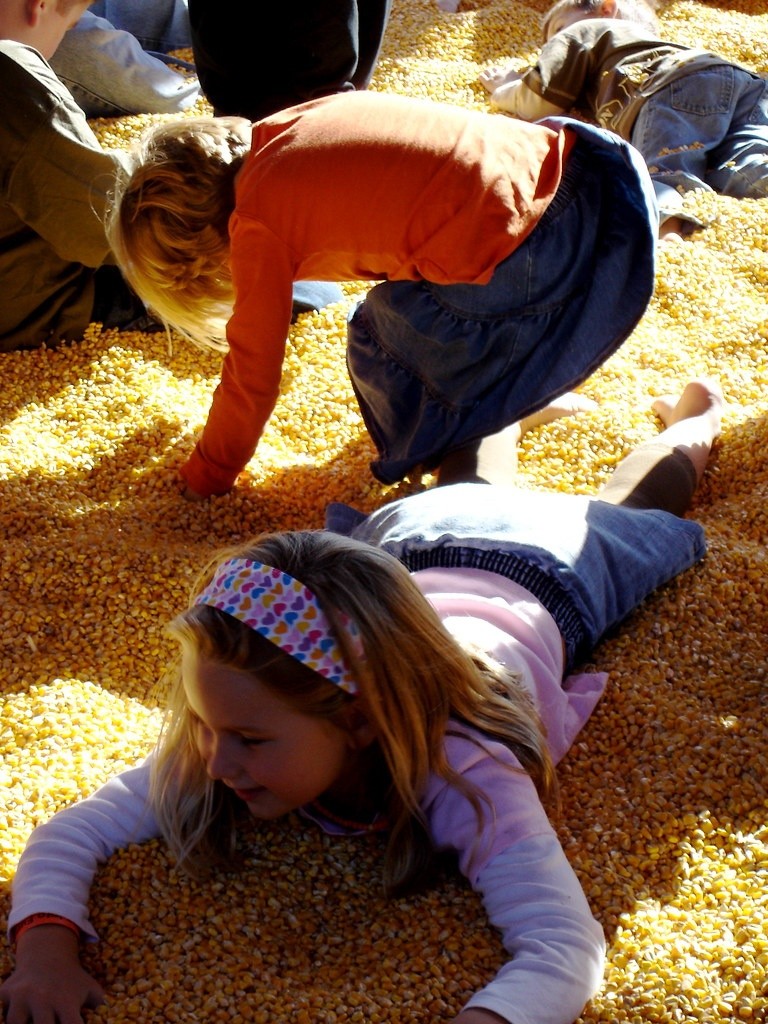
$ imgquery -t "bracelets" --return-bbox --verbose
[16,912,79,940]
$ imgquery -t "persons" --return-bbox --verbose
[478,0,768,244]
[0,380,736,1024]
[0,0,390,350]
[108,91,661,500]
